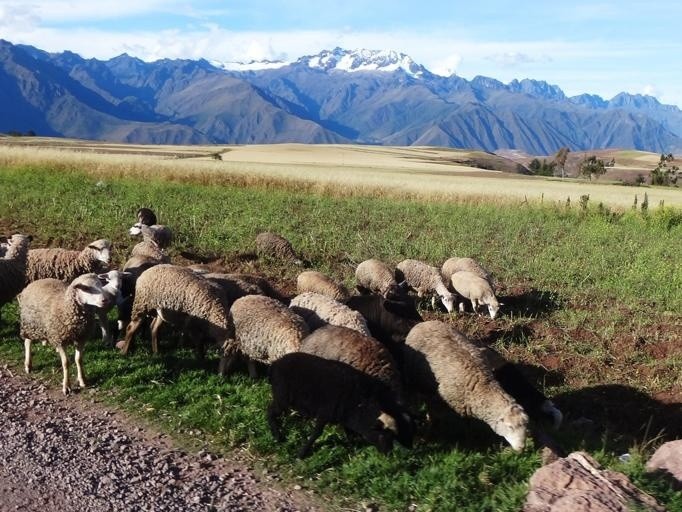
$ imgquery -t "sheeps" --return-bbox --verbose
[1,237,114,288]
[257,234,305,267]
[441,255,490,288]
[298,324,415,447]
[289,291,371,337]
[129,222,175,250]
[229,293,308,379]
[1,233,30,307]
[16,273,107,395]
[137,205,159,227]
[132,241,166,263]
[123,254,161,306]
[297,271,350,304]
[449,269,504,322]
[204,272,274,296]
[401,318,531,458]
[395,255,459,315]
[264,350,400,459]
[119,262,241,378]
[347,294,401,356]
[85,270,123,345]
[353,259,402,300]
